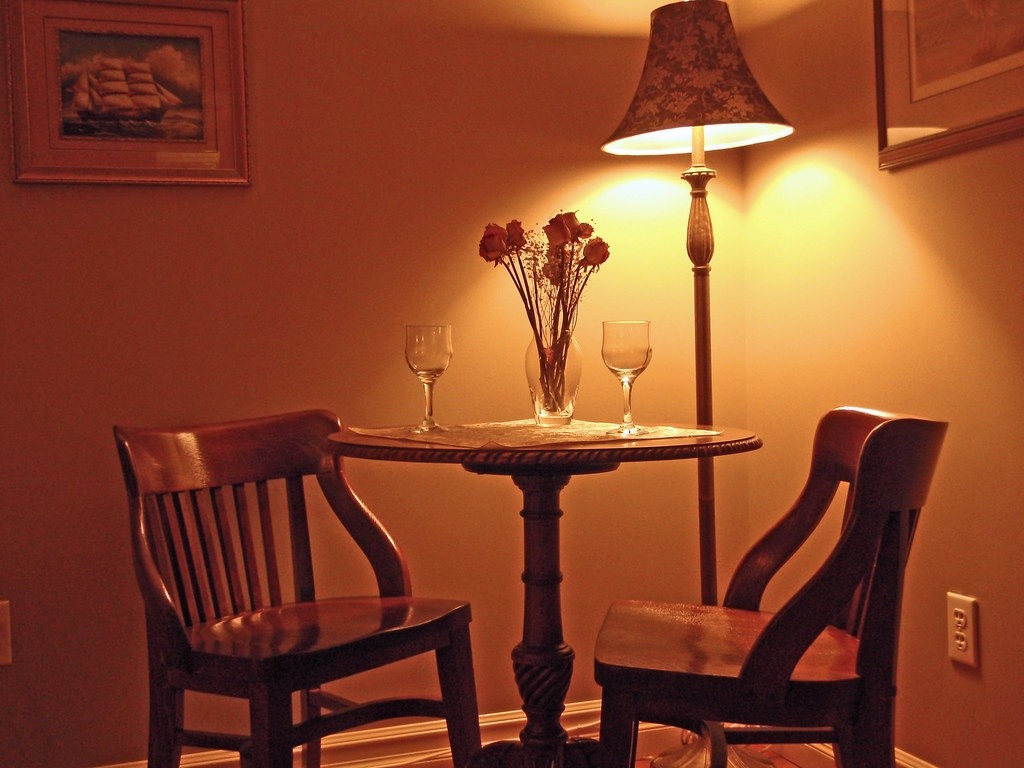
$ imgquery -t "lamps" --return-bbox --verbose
[601,0,795,768]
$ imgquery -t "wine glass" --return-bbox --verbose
[405,324,454,435]
[601,320,653,437]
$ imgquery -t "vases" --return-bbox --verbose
[524,327,585,425]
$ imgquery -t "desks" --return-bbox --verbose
[325,425,764,768]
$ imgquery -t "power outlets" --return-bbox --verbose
[946,590,976,670]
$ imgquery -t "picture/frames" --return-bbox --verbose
[0,0,250,187]
[873,0,1023,169]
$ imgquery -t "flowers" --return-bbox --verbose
[477,209,609,410]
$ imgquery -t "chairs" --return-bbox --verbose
[594,407,950,768]
[114,408,481,768]
